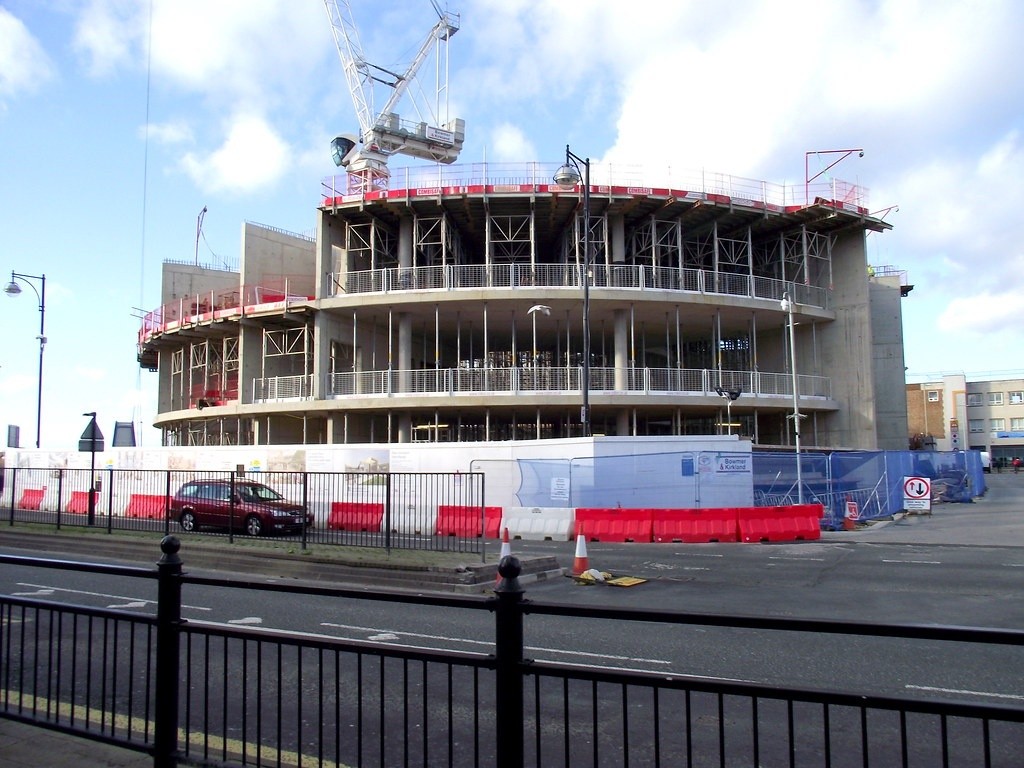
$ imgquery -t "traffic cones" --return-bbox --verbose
[838,495,859,531]
[495,526,511,584]
[565,521,591,577]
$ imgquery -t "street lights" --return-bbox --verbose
[82,411,97,526]
[551,142,590,437]
[923,382,933,436]
[781,290,805,505]
[714,386,742,435]
[3,269,45,448]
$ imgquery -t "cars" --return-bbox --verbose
[170,478,315,536]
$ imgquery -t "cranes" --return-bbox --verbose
[320,0,467,195]
